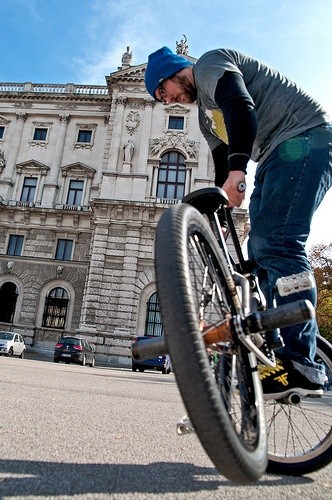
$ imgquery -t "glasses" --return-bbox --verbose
[159,80,170,106]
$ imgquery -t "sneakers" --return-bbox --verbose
[235,358,323,400]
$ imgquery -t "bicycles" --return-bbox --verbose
[135,180,332,483]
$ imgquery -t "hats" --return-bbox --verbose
[144,46,195,102]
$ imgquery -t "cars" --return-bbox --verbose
[131,334,172,375]
[53,336,96,367]
[0,331,26,359]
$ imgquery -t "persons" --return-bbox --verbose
[146,46,332,397]
[123,140,135,162]
[121,33,189,67]
